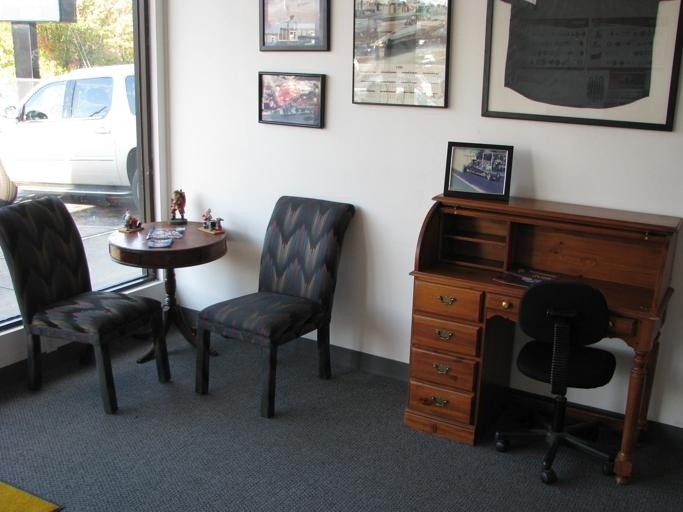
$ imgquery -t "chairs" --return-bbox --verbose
[195,195,354,419]
[0,198,170,414]
[495,281,616,484]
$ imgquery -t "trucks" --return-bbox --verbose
[463,152,505,181]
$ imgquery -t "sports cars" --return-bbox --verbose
[359,14,444,52]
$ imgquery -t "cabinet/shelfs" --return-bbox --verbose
[403,193,682,485]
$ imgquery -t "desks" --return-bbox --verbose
[108,221,228,363]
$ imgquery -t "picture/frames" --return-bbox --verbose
[352,0,451,108]
[258,71,325,128]
[259,0,330,51]
[481,0,683,131]
[443,141,514,201]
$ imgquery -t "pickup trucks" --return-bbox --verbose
[0,64,142,214]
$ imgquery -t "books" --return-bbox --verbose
[491,266,557,290]
[145,225,186,240]
